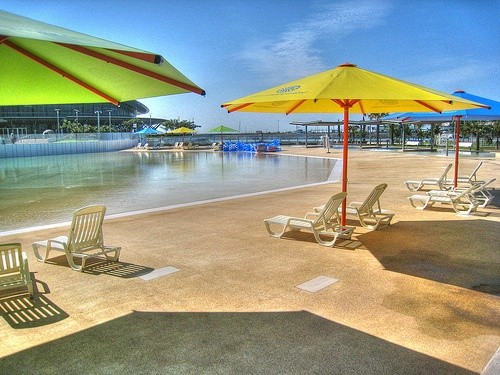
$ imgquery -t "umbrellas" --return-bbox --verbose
[132,127,162,146]
[221,62,490,231]
[379,91,500,189]
[165,127,199,144]
[207,125,240,143]
[0,10,206,108]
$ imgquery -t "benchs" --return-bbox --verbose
[454,142,473,150]
[404,141,419,148]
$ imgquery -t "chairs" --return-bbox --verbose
[404,162,496,215]
[132,142,183,150]
[262,192,355,247]
[32,205,121,272]
[305,183,395,231]
[0,243,34,300]
